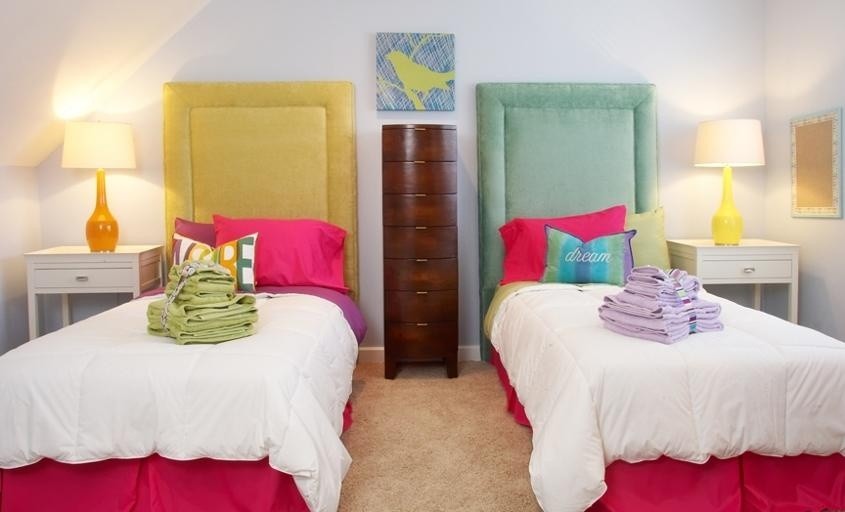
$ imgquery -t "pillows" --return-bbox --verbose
[162,228,263,296]
[209,209,353,296]
[494,198,630,287]
[533,220,640,289]
[170,214,215,246]
[622,203,673,272]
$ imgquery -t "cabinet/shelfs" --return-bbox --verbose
[376,121,464,384]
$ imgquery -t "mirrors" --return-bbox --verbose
[786,104,843,224]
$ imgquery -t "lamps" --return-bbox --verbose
[57,115,145,254]
[691,115,767,246]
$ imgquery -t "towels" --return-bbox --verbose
[138,257,262,349]
[596,260,726,348]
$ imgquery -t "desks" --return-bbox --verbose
[662,233,802,326]
[21,242,162,343]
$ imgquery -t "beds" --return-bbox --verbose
[472,80,845,510]
[0,75,364,512]
